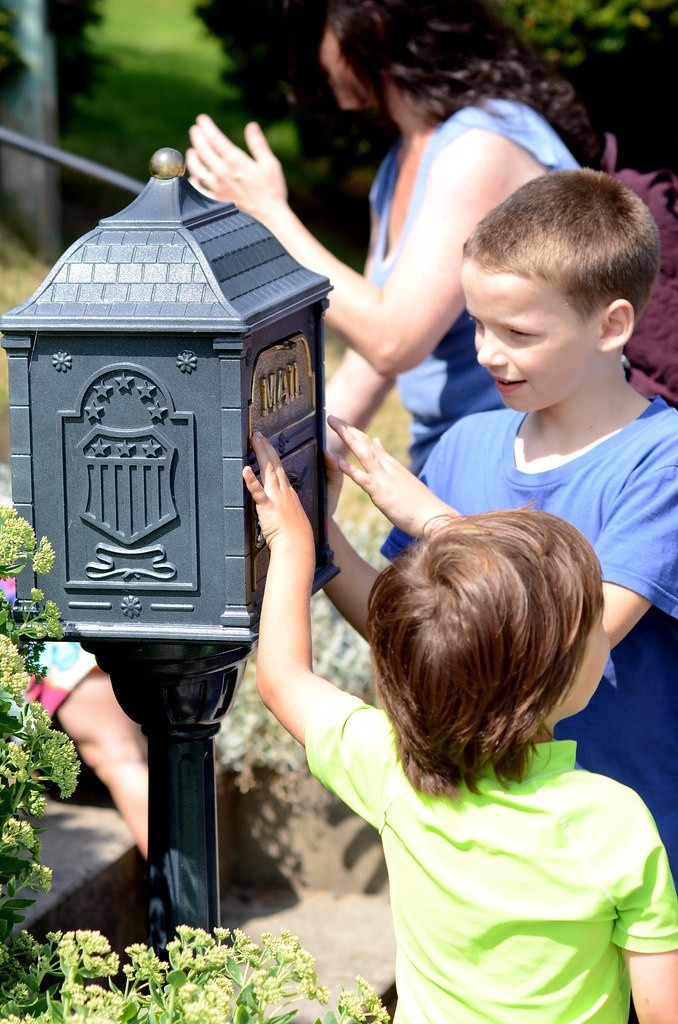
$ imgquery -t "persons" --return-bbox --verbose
[322,169,678,895]
[186,0,602,477]
[240,427,678,1024]
[0,345,149,986]
